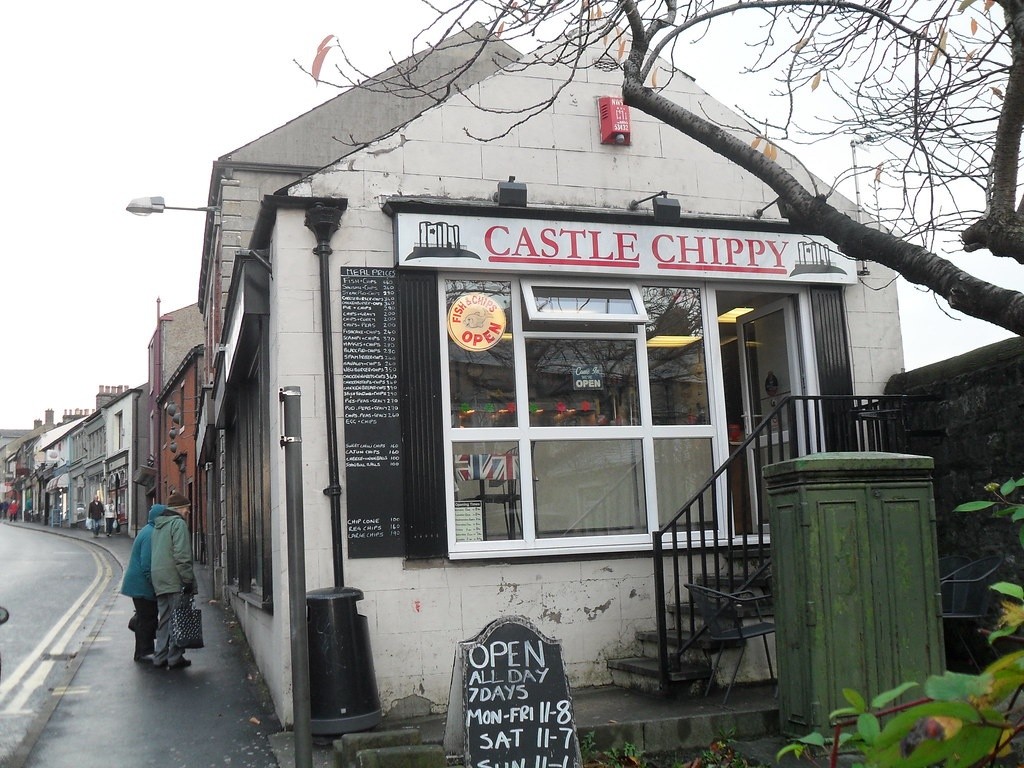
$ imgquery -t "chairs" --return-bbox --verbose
[683,583,776,704]
[938,555,1005,675]
[476,442,535,538]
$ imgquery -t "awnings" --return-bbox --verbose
[46,473,68,494]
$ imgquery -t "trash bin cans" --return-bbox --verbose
[307,585,382,734]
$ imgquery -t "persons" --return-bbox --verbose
[9,499,17,521]
[88,495,105,537]
[151,491,198,668]
[120,504,169,663]
[104,498,117,537]
[2,500,9,519]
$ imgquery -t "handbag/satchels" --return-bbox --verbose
[112,519,117,528]
[171,586,204,650]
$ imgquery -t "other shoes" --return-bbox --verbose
[107,532,111,537]
[168,658,191,671]
[128,622,137,632]
[134,652,153,664]
[94,534,98,538]
[154,660,170,669]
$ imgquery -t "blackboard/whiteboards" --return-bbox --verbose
[441,615,583,768]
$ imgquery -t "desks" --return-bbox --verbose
[452,453,539,540]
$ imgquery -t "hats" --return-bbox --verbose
[167,491,192,511]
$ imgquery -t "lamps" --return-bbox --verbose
[126,195,221,215]
[629,191,682,220]
[753,196,789,218]
[493,175,527,207]
[166,389,201,452]
